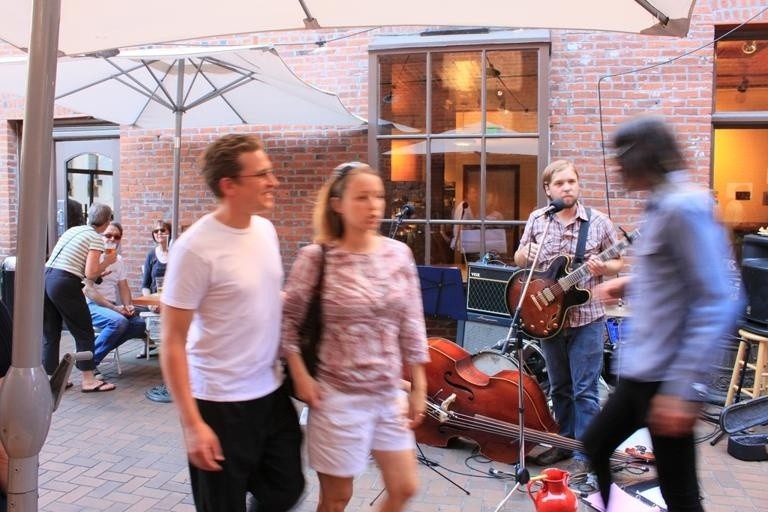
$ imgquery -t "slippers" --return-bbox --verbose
[81,381,116,392]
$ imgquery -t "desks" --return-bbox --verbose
[131,291,160,305]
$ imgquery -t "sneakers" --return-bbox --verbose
[136,344,156,359]
[91,368,106,380]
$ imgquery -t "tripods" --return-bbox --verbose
[490,222,588,512]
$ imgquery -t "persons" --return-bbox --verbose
[723,186,756,269]
[512,160,620,478]
[448,184,504,264]
[42,202,118,392]
[133,220,172,359]
[278,161,430,512]
[575,114,746,512]
[156,132,309,512]
[83,222,145,380]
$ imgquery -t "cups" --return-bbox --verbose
[103,242,119,263]
[155,277,166,295]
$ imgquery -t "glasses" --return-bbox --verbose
[153,228,165,233]
[330,162,356,189]
[228,167,274,181]
[105,234,122,240]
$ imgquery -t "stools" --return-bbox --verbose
[93,325,122,375]
[724,327,768,432]
[140,311,160,361]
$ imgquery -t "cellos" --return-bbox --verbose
[403,337,656,466]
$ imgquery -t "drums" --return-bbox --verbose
[472,338,552,400]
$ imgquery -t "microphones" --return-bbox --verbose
[534,198,565,219]
[395,204,415,217]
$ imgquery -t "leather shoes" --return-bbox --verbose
[535,445,574,466]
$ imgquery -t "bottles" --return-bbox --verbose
[527,467,578,512]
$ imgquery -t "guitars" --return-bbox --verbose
[504,228,637,340]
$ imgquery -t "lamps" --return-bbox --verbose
[383,94,400,104]
[738,75,749,92]
[742,40,757,54]
[485,67,501,76]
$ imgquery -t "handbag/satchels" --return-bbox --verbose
[284,292,321,402]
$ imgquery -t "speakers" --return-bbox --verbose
[737,234,768,337]
[456,312,518,359]
